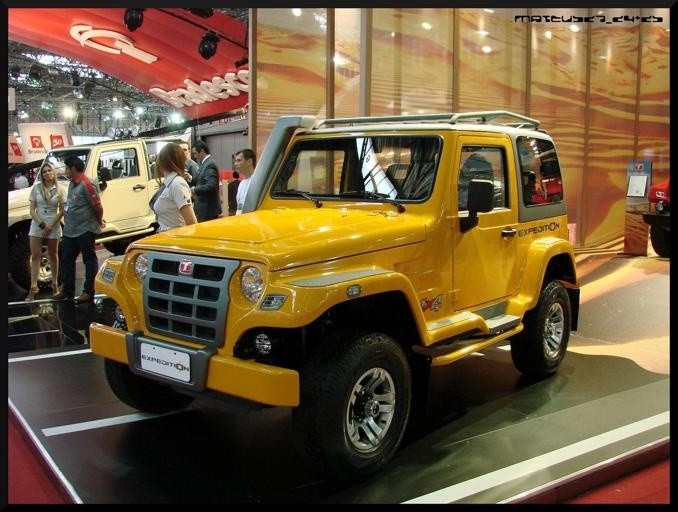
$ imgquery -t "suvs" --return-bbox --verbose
[8,137,173,292]
[89,110,579,484]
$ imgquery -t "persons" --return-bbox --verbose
[25,162,65,301]
[150,142,198,232]
[7,167,38,191]
[179,142,199,210]
[185,139,222,221]
[235,149,257,213]
[227,171,241,216]
[51,156,106,302]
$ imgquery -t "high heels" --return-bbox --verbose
[24,286,40,301]
[51,283,60,296]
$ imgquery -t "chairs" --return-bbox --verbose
[110,154,157,179]
[383,153,495,211]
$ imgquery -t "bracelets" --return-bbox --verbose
[38,222,46,229]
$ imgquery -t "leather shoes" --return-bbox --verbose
[52,292,94,304]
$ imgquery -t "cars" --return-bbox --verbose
[643,179,671,257]
